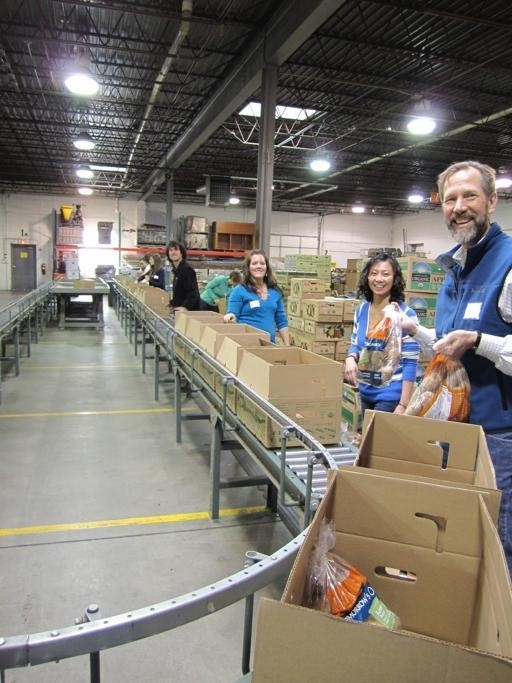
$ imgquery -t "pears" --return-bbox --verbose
[328,327,335,336]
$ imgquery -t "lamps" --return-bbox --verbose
[351,175,512,213]
[64,48,102,198]
[225,101,436,205]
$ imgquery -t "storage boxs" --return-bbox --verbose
[72,278,97,288]
[338,408,504,529]
[112,252,445,447]
[247,466,511,682]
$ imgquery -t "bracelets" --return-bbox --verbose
[398,400,408,409]
[471,329,482,353]
[345,354,357,361]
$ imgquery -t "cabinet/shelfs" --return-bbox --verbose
[211,220,256,250]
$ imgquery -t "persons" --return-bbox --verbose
[342,253,421,432]
[59,255,66,272]
[224,250,291,345]
[390,160,510,579]
[57,259,61,272]
[136,240,244,312]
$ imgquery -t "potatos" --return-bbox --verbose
[355,337,399,386]
[405,368,470,419]
